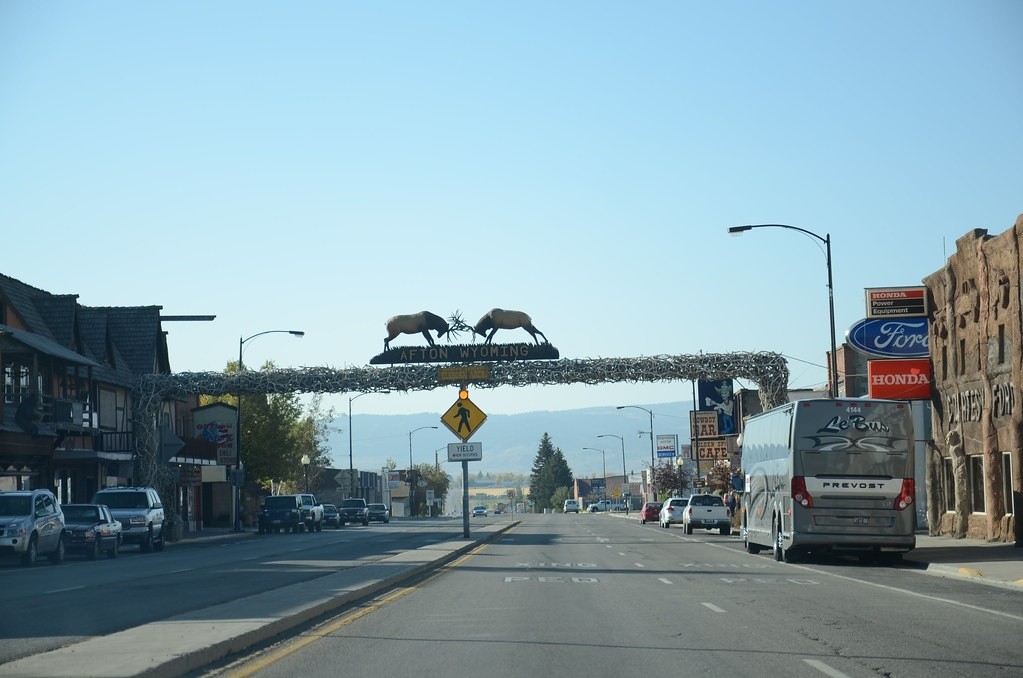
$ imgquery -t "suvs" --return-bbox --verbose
[0,489,67,569]
[258,495,305,534]
[91,484,166,554]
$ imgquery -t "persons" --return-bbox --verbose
[727,492,736,517]
[945,430,962,459]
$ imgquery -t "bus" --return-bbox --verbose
[731,398,919,563]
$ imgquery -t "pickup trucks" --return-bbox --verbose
[588,500,626,514]
[683,494,731,536]
[298,493,324,533]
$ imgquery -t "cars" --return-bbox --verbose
[321,502,340,527]
[59,504,122,562]
[472,506,489,517]
[639,497,694,531]
[368,504,390,524]
[338,498,370,527]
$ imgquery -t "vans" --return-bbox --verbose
[563,499,580,514]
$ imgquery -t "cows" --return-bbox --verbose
[384,310,449,353]
[473,308,548,345]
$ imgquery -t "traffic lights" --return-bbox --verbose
[459,389,469,400]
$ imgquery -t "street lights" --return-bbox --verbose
[582,404,683,515]
[409,426,439,515]
[349,390,391,499]
[729,223,840,397]
[231,330,306,534]
